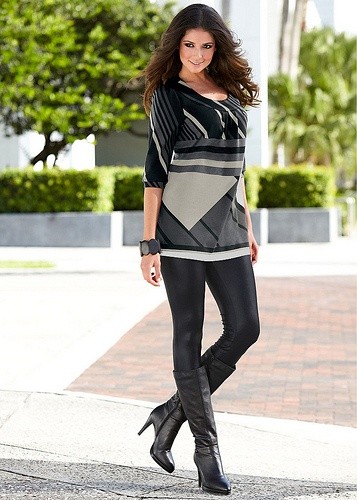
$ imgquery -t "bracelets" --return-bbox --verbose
[138,238,162,258]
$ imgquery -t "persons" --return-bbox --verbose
[134,2,264,497]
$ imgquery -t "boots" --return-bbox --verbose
[172,365,230,494]
[138,343,237,473]
